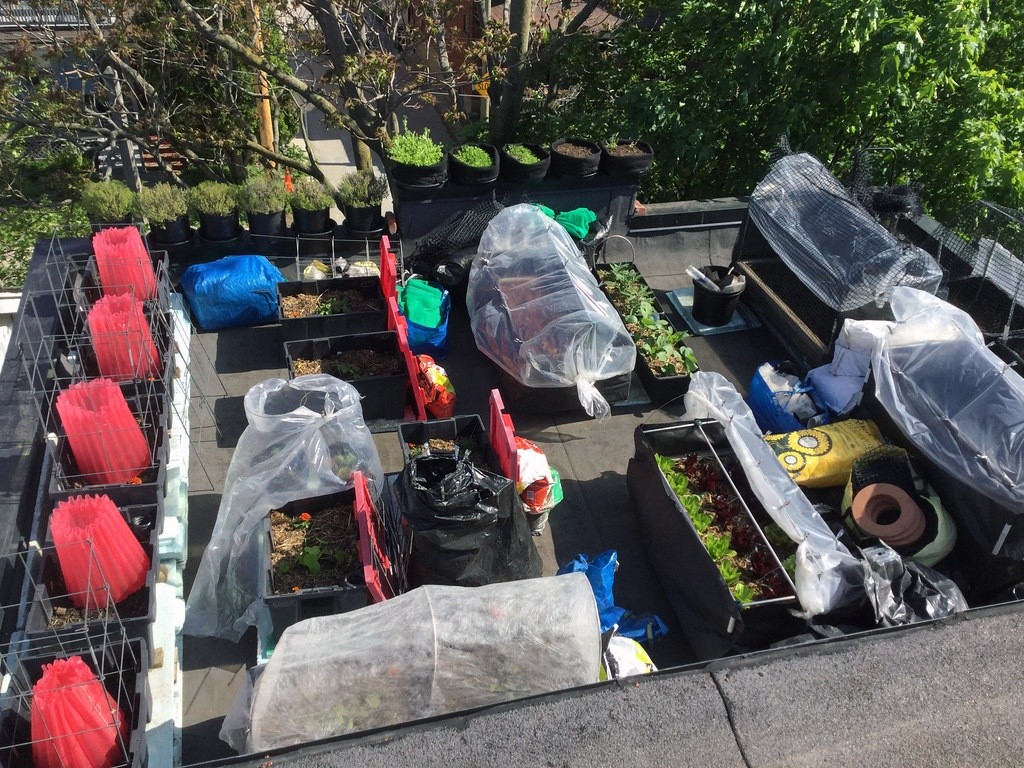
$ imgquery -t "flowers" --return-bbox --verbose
[278,511,343,593]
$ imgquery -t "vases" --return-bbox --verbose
[551,138,603,189]
[263,485,371,638]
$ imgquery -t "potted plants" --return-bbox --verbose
[635,418,806,639]
[451,141,503,193]
[380,112,448,197]
[282,329,409,420]
[593,258,703,403]
[501,143,552,191]
[81,169,389,254]
[601,133,654,187]
[275,275,389,345]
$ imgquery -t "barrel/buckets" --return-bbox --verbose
[692,265,747,326]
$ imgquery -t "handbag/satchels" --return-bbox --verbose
[744,357,828,436]
[556,548,669,645]
[398,280,452,360]
